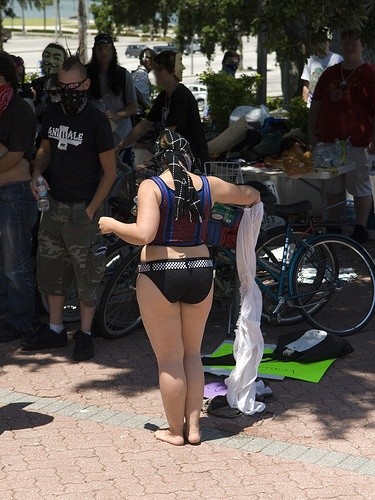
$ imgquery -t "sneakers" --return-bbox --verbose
[73,330,93,360]
[22,326,67,350]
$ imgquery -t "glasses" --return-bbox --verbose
[59,78,88,89]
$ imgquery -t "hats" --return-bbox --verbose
[95,34,112,45]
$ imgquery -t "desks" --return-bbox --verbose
[241,159,357,234]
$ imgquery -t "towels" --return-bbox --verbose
[224,202,267,416]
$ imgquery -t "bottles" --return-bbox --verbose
[314,140,345,168]
[96,99,106,114]
[36,177,49,211]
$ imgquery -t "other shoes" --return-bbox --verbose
[352,226,369,245]
[327,227,342,235]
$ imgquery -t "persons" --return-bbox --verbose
[222,46,240,77]
[307,28,375,248]
[0,32,210,363]
[301,27,344,110]
[98,127,261,445]
